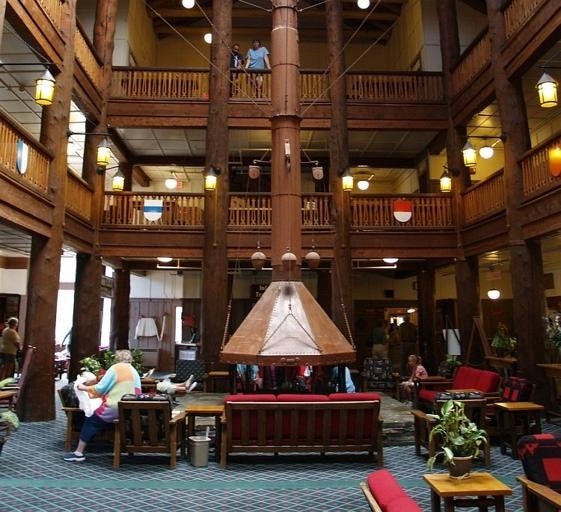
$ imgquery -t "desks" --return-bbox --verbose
[209,371,230,392]
[185,405,225,463]
[495,402,544,459]
[423,473,512,512]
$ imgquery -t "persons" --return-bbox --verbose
[0,317,22,381]
[230,43,243,98]
[237,363,259,392]
[245,38,272,98]
[400,353,429,406]
[157,374,198,395]
[74,371,104,417]
[63,349,142,461]
[331,365,355,393]
[370,318,393,363]
[400,316,417,369]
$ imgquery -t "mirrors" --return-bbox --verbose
[175,305,201,346]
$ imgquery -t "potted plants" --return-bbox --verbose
[427,399,489,476]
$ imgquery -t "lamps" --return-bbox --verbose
[487,266,500,300]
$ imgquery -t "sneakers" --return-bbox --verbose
[185,381,198,394]
[184,374,195,389]
[63,453,85,462]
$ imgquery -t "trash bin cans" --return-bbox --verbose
[188,436,211,467]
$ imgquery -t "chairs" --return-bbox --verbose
[360,357,401,401]
[168,360,208,393]
[480,377,537,438]
[411,390,491,467]
[57,378,188,469]
[0,345,36,421]
[516,434,561,512]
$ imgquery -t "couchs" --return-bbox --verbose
[220,393,385,468]
[360,469,423,512]
[414,367,503,414]
